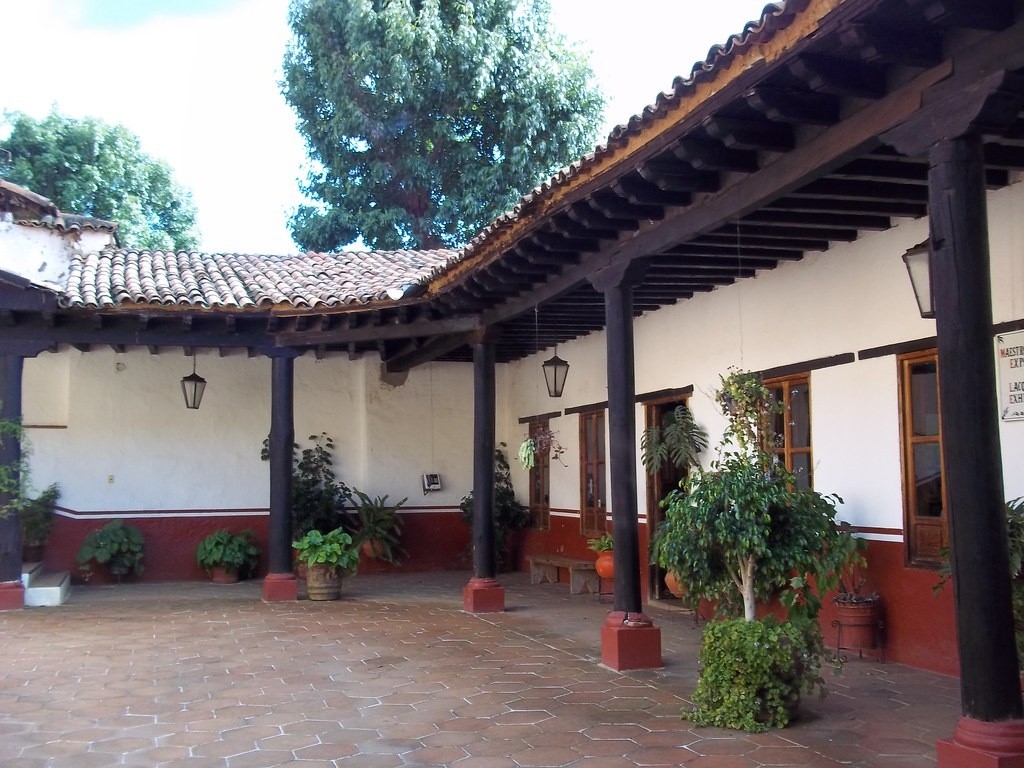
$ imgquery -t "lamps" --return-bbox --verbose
[19,483,59,562]
[180,348,207,409]
[542,344,570,398]
[901,238,936,319]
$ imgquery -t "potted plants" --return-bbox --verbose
[334,488,409,567]
[198,529,259,583]
[76,520,145,583]
[585,532,616,578]
[648,446,845,733]
[261,431,353,579]
[460,442,529,574]
[832,591,883,648]
[293,527,359,601]
